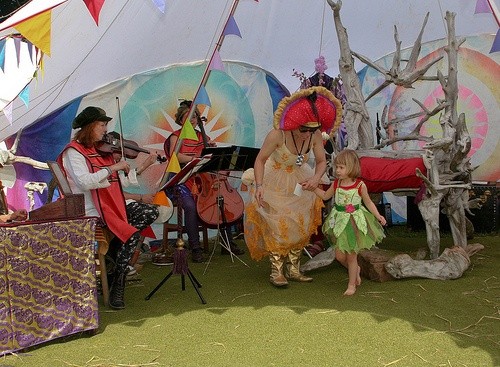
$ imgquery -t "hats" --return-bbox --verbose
[175,100,200,125]
[72,107,112,130]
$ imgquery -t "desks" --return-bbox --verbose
[0,216,108,357]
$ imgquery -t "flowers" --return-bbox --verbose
[291,55,349,153]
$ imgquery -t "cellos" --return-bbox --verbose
[183,107,245,264]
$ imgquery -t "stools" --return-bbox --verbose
[163,202,209,254]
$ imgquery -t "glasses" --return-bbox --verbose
[296,126,320,133]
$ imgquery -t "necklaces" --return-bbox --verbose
[291,131,305,166]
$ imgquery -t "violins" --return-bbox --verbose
[93,130,168,165]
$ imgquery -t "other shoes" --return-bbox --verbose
[220,243,245,255]
[192,248,216,263]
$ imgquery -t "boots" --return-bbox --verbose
[108,266,130,310]
[268,249,312,286]
[105,236,123,265]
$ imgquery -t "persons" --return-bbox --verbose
[62,106,158,310]
[164,100,245,262]
[254,87,336,287]
[300,149,387,296]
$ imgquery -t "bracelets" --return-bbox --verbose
[257,184,263,188]
